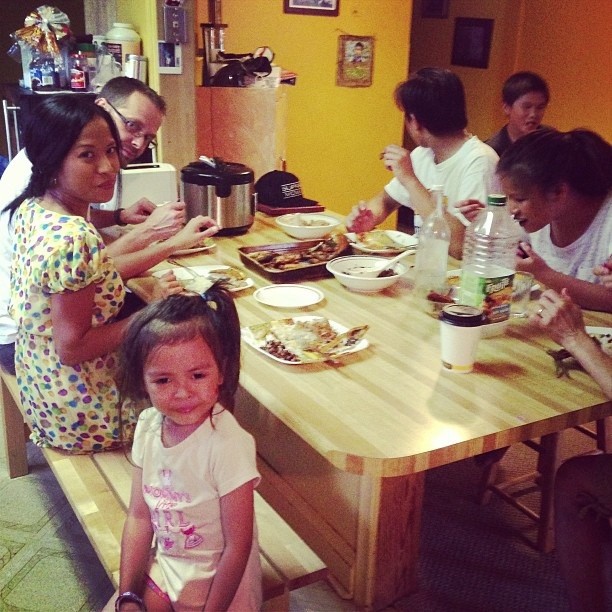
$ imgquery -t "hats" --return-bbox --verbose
[254,170,319,207]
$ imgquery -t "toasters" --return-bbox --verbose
[100,164,180,208]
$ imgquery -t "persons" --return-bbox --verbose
[0,75,186,380]
[9,91,220,455]
[347,41,367,65]
[103,278,263,612]
[482,71,550,158]
[350,66,504,262]
[454,127,612,314]
[592,257,611,288]
[533,286,612,612]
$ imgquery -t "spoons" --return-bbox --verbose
[363,249,419,274]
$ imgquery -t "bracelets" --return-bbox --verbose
[115,592,149,612]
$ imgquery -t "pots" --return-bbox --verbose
[180,157,257,236]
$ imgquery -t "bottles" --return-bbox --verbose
[460,193,521,340]
[108,22,140,72]
[415,190,450,288]
[71,55,90,91]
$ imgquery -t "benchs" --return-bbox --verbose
[1,360,329,612]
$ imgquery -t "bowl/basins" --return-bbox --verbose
[325,255,409,292]
[275,213,342,240]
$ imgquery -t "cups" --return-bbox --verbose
[439,304,487,373]
[510,271,534,317]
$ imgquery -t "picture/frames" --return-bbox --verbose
[283,0,339,18]
[105,202,612,600]
[448,17,495,68]
[334,35,374,86]
[420,1,449,19]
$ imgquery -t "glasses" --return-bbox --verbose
[106,98,158,149]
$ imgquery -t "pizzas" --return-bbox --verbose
[245,232,350,273]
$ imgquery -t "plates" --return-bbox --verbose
[151,263,254,296]
[253,283,324,310]
[341,230,419,253]
[449,264,543,302]
[154,238,216,254]
[241,316,369,366]
